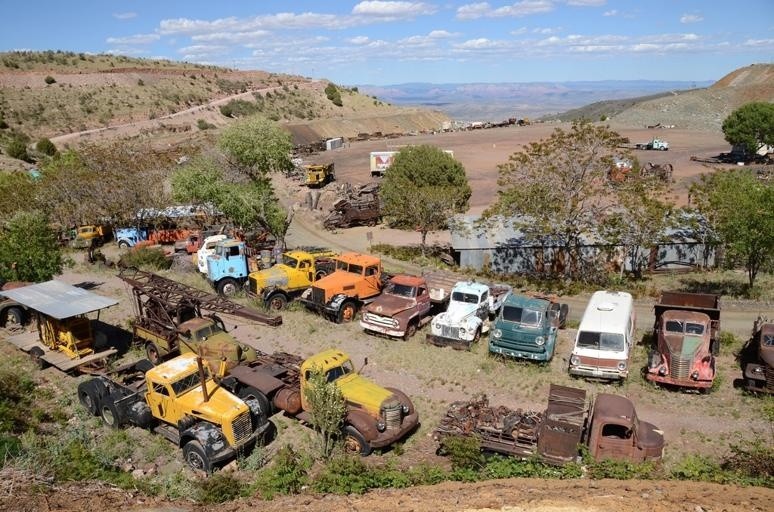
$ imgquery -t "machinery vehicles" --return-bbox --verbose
[230,349,420,457]
[116,264,281,378]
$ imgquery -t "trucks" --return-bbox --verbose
[433,382,665,468]
[488,292,567,362]
[306,162,336,188]
[72,223,202,254]
[426,279,511,350]
[359,275,448,341]
[633,139,670,152]
[296,252,391,323]
[3,278,276,473]
[248,250,337,312]
[644,292,722,394]
[1,281,31,330]
[738,324,773,397]
[195,235,273,297]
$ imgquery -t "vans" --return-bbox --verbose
[569,292,635,383]
